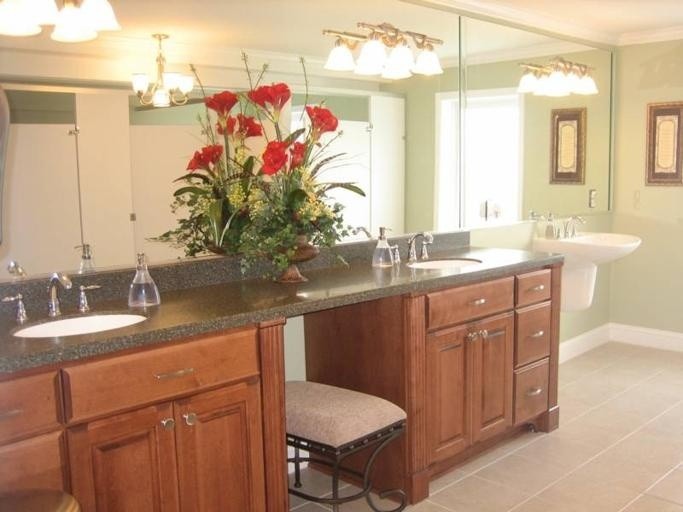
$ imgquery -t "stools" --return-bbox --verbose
[0,488,82,512]
[285,381,407,512]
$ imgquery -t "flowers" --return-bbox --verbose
[143,91,265,258]
[212,50,371,282]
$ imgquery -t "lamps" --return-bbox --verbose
[545,57,598,95]
[131,34,194,108]
[0,0,122,43]
[355,21,444,78]
[517,63,570,97]
[317,28,410,79]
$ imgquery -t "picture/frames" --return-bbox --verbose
[550,108,585,184]
[645,101,683,188]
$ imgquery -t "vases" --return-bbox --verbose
[267,243,321,282]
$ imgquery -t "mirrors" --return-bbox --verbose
[0,0,613,282]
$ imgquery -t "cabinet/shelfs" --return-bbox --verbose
[305,260,563,505]
[0,318,291,512]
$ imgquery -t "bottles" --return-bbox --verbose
[127,251,160,308]
[78,245,94,274]
[372,227,393,267]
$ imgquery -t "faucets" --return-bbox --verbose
[409,231,434,261]
[47,271,72,317]
[7,259,26,277]
[564,215,587,238]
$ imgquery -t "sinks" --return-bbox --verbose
[12,313,147,338]
[407,259,483,270]
[553,232,642,264]
